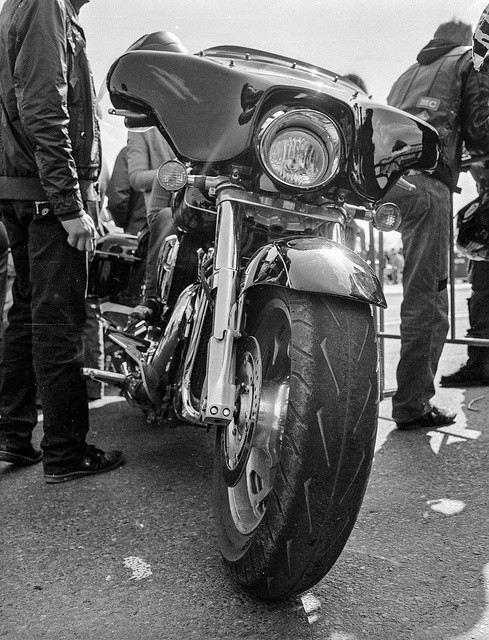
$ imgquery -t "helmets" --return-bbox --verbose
[456,192,489,262]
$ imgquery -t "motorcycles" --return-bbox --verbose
[79,30,443,602]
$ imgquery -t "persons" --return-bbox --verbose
[106,145,150,235]
[386,22,488,432]
[0,157,109,405]
[0,0,126,485]
[441,149,488,388]
[358,244,405,284]
[126,125,197,320]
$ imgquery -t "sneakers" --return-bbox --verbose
[0,436,43,464]
[44,446,126,483]
[395,407,457,429]
[130,299,160,319]
[440,365,488,387]
